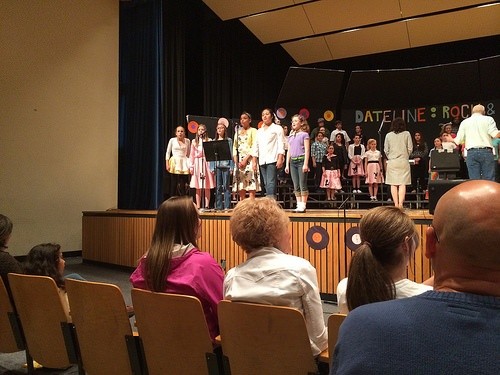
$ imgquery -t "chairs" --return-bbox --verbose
[0,271,350,375]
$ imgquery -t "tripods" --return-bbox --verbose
[198,140,233,212]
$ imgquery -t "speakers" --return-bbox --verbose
[428,179,468,215]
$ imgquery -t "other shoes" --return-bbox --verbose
[295,202,307,213]
[352,189,362,193]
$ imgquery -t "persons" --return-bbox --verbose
[409,130,429,193]
[210,123,234,210]
[189,124,213,209]
[282,114,310,212]
[24,243,135,323]
[129,195,225,341]
[319,144,342,201]
[330,180,500,375]
[310,117,365,194]
[232,112,262,201]
[0,214,28,303]
[336,206,434,314]
[453,104,500,181]
[428,121,460,180]
[165,126,191,197]
[222,197,328,357]
[383,117,414,208]
[252,107,285,198]
[363,138,385,202]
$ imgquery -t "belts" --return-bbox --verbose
[292,157,304,160]
[467,147,492,151]
